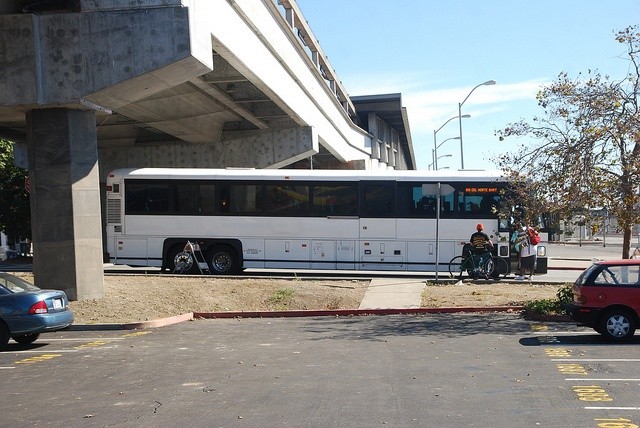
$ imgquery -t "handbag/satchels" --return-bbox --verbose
[526,228,540,245]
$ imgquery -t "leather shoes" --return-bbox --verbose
[484,273,490,280]
[474,277,478,280]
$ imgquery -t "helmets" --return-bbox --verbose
[476,224,483,230]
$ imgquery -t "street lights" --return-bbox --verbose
[432,135,462,169]
[459,80,496,168]
[434,114,470,170]
[428,154,450,169]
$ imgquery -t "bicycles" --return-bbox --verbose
[448,243,508,280]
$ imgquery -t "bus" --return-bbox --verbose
[106,166,546,277]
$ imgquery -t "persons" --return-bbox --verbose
[510,217,538,279]
[468,223,492,281]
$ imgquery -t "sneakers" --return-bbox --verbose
[514,275,524,280]
[528,276,533,280]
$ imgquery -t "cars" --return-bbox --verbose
[0,271,74,348]
[567,258,640,341]
[0,246,19,264]
